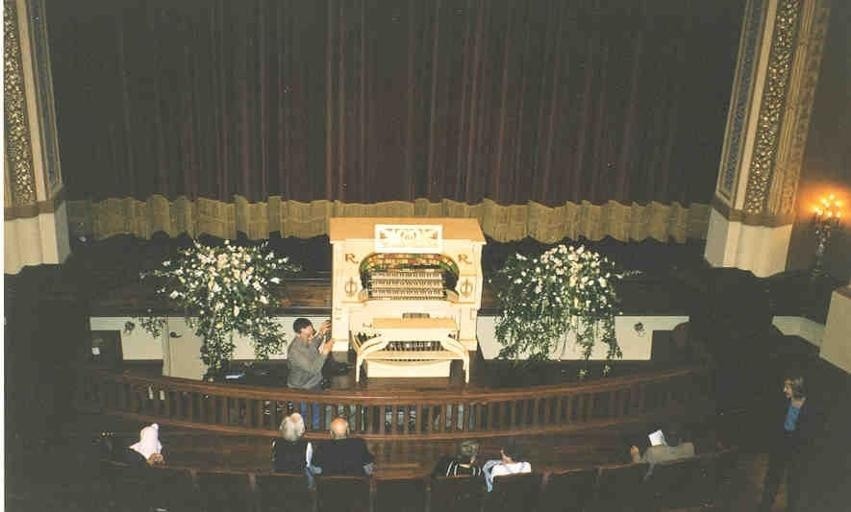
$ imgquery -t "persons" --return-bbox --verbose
[760,376,815,512]
[630,431,694,463]
[433,441,483,478]
[271,413,313,473]
[313,418,375,476]
[483,443,532,493]
[287,317,334,388]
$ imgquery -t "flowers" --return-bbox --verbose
[491,241,649,383]
[120,233,305,383]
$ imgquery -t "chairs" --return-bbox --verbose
[255,472,314,509]
[101,457,151,509]
[197,469,262,510]
[489,471,543,510]
[430,475,482,510]
[697,444,742,510]
[542,467,597,510]
[642,455,701,510]
[374,476,426,509]
[596,461,649,510]
[317,473,372,511]
[152,466,204,509]
